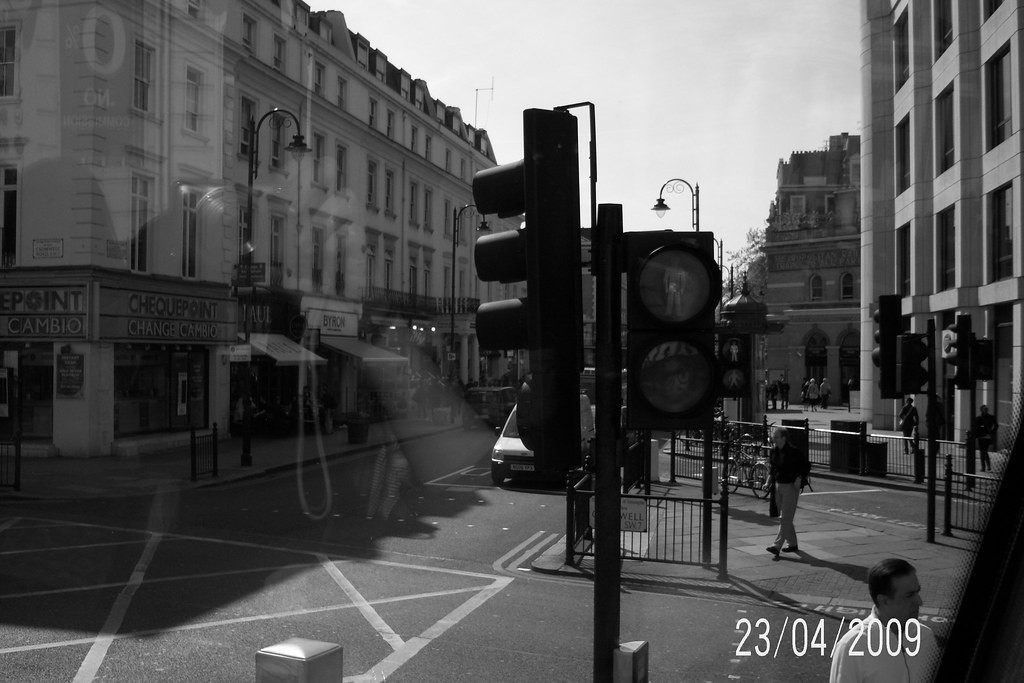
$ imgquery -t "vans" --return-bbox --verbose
[490,395,594,484]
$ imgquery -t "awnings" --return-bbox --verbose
[237,332,329,367]
[320,336,409,367]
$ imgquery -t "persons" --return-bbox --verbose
[898,397,920,455]
[975,405,999,472]
[230,385,335,439]
[762,427,810,555]
[766,374,832,413]
[412,374,529,425]
[926,394,945,456]
[829,558,941,683]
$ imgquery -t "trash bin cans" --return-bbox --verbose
[866,441,888,476]
[782,419,809,457]
[348,416,369,443]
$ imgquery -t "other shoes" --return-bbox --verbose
[766,546,780,555]
[904,446,915,454]
[782,545,798,552]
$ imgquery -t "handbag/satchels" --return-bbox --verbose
[899,418,903,426]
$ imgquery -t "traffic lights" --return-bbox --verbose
[903,334,929,390]
[719,337,748,397]
[947,313,973,392]
[626,230,715,429]
[872,294,901,398]
[471,109,582,454]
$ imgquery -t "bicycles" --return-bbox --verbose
[678,404,772,499]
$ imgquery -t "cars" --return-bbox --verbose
[463,385,519,426]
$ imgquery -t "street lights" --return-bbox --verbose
[653,178,713,570]
[448,205,495,424]
[237,109,313,461]
